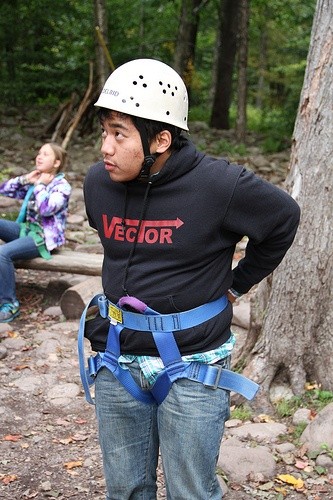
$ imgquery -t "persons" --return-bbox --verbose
[0,142,72,323]
[83,58,301,500]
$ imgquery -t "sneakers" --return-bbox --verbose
[0,300,21,324]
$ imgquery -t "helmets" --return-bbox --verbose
[93,58,190,133]
